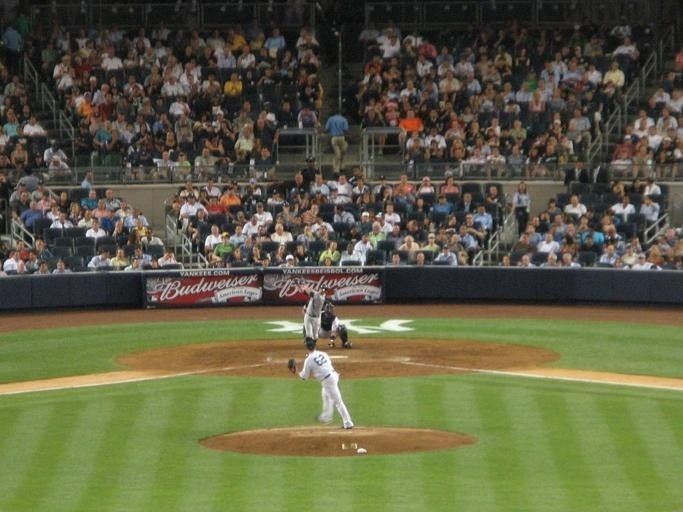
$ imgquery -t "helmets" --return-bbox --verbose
[321,284,328,290]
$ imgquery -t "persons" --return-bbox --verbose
[289,284,354,430]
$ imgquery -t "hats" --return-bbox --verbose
[442,243,448,248]
[362,211,385,218]
[20,179,44,186]
[286,255,294,262]
[427,232,436,239]
[221,232,230,238]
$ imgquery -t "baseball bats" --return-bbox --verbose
[292,277,304,292]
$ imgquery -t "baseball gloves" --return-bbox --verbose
[288,359,296,372]
[327,340,336,348]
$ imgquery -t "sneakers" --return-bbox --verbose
[343,421,354,429]
[318,416,332,423]
[343,342,353,348]
[304,337,317,351]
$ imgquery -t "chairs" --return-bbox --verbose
[13,179,669,269]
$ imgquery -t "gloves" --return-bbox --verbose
[328,336,336,348]
